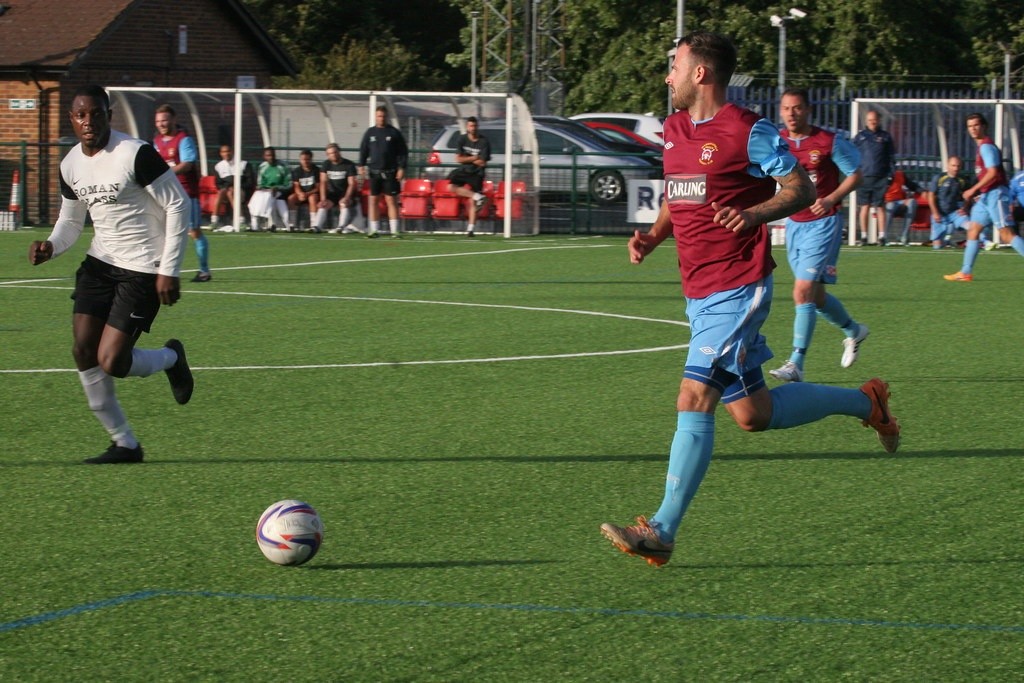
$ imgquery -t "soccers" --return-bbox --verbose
[254,499,325,568]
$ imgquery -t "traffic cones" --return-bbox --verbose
[8,170,20,211]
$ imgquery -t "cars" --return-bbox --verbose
[562,122,669,154]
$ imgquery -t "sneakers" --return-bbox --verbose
[769,360,803,382]
[858,377,902,453]
[163,339,194,404]
[840,324,871,368]
[207,194,490,240]
[83,440,144,464]
[600,515,675,566]
[943,271,972,281]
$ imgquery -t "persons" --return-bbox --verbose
[357,105,409,237]
[309,142,356,233]
[27,83,195,461]
[150,105,212,278]
[768,85,872,382]
[884,166,925,244]
[851,109,895,246]
[599,30,898,564]
[288,149,320,231]
[447,116,490,238]
[247,147,293,233]
[207,143,253,228]
[926,110,1024,281]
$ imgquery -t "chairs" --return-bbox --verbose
[178,176,526,232]
[911,193,931,231]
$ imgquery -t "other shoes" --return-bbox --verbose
[191,272,212,282]
[877,238,886,246]
[856,238,867,247]
[984,243,997,251]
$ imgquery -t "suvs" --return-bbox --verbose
[417,115,663,208]
[565,112,667,147]
